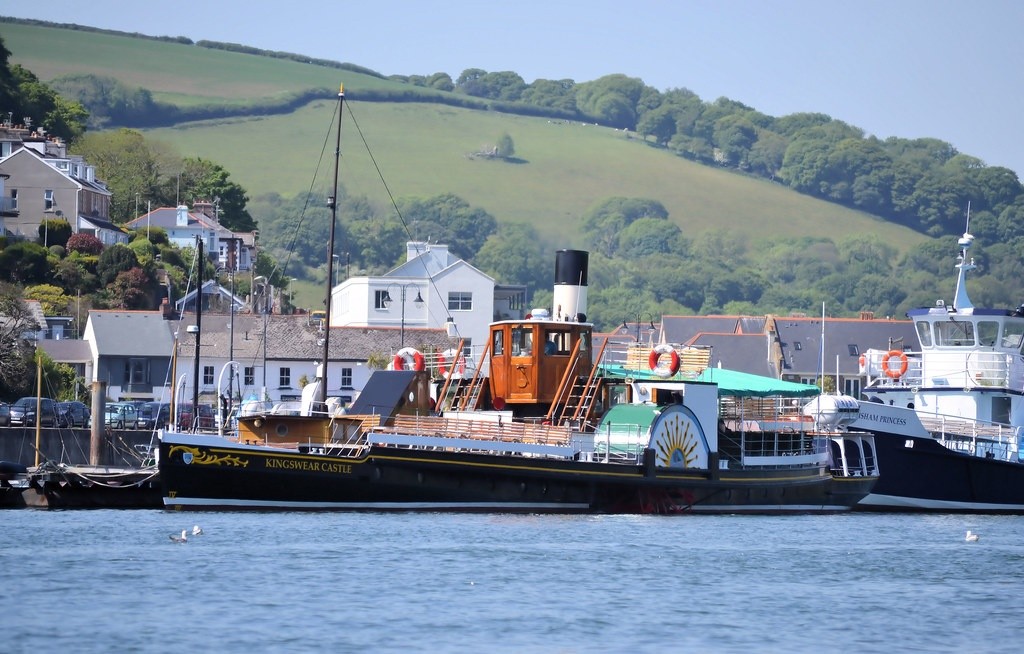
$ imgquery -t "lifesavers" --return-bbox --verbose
[394,346,425,371]
[648,343,681,379]
[881,349,908,377]
[438,348,468,379]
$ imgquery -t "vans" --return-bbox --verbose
[175,402,216,433]
[136,401,170,430]
[104,402,138,430]
[9,397,60,428]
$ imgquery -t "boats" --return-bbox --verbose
[1,80,1022,515]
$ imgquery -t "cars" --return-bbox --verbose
[55,401,91,428]
